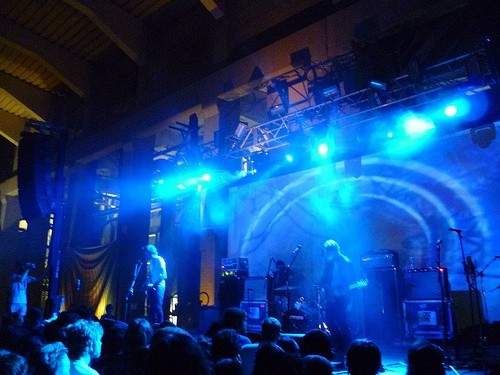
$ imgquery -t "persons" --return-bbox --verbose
[345,338,381,375]
[406,342,446,375]
[102,304,115,319]
[321,239,355,351]
[141,245,167,323]
[8,258,43,321]
[0,307,334,375]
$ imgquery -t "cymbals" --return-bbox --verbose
[272,286,302,291]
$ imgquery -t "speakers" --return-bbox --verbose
[198,307,217,332]
[243,276,268,300]
[404,268,449,300]
[362,269,405,340]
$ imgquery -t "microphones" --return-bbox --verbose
[272,257,277,263]
[448,228,462,232]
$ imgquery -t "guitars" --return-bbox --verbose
[141,277,157,291]
[319,278,370,306]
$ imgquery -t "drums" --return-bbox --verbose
[283,308,308,331]
